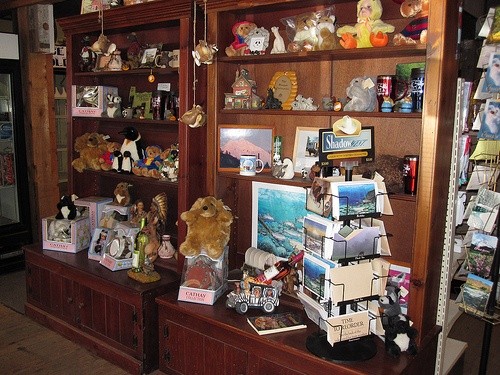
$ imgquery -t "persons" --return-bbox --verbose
[94,230,108,256]
[130,191,167,262]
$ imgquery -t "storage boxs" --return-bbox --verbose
[178,245,229,307]
[71,84,118,118]
[72,196,113,238]
[41,215,91,254]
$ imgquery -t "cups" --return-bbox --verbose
[239,155,264,177]
[322,97,333,110]
[152,90,165,120]
[377,75,407,112]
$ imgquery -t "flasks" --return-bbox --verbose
[404,155,417,195]
[411,68,425,112]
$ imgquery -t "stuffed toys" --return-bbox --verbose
[112,182,133,207]
[286,12,341,52]
[55,193,82,220]
[378,286,401,318]
[179,105,206,127]
[393,0,430,46]
[192,40,218,67]
[106,94,121,118]
[90,34,116,56]
[336,0,396,49]
[179,195,234,259]
[225,21,257,57]
[71,126,179,184]
[48,219,71,241]
[343,75,377,112]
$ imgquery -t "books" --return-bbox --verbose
[246,312,308,336]
[455,7,500,318]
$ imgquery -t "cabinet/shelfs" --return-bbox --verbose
[19,0,469,375]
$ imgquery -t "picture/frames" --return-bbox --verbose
[217,124,275,172]
[292,125,323,174]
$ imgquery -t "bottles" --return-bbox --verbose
[167,91,175,109]
[254,251,304,285]
[273,136,282,164]
[132,218,148,272]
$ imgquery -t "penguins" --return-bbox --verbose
[110,127,147,175]
